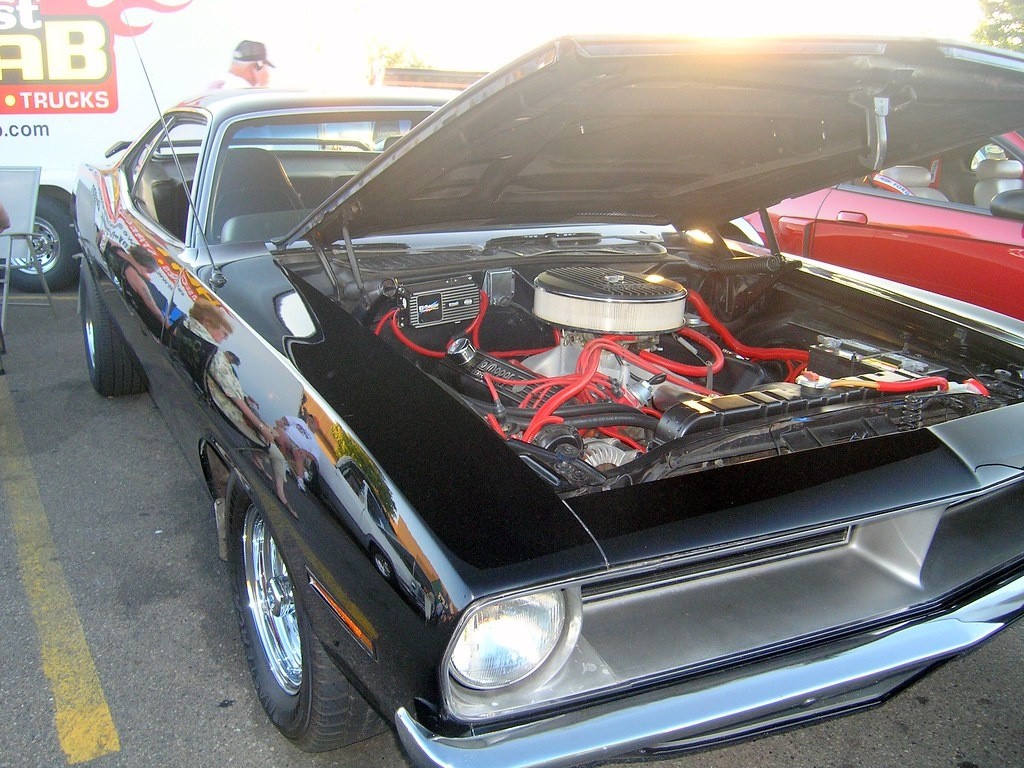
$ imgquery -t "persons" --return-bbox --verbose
[203,41,276,99]
[0,201,11,375]
[98,236,321,519]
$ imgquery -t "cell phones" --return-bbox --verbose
[256,62,264,72]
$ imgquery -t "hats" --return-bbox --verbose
[234,40,276,68]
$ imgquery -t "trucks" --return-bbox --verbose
[1,0,385,294]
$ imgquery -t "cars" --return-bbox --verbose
[76,37,1024,768]
[743,129,1024,320]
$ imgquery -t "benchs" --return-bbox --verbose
[925,160,961,203]
[149,151,385,244]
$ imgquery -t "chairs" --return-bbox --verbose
[973,159,1023,208]
[878,166,951,203]
[214,147,308,241]
[0,164,60,336]
